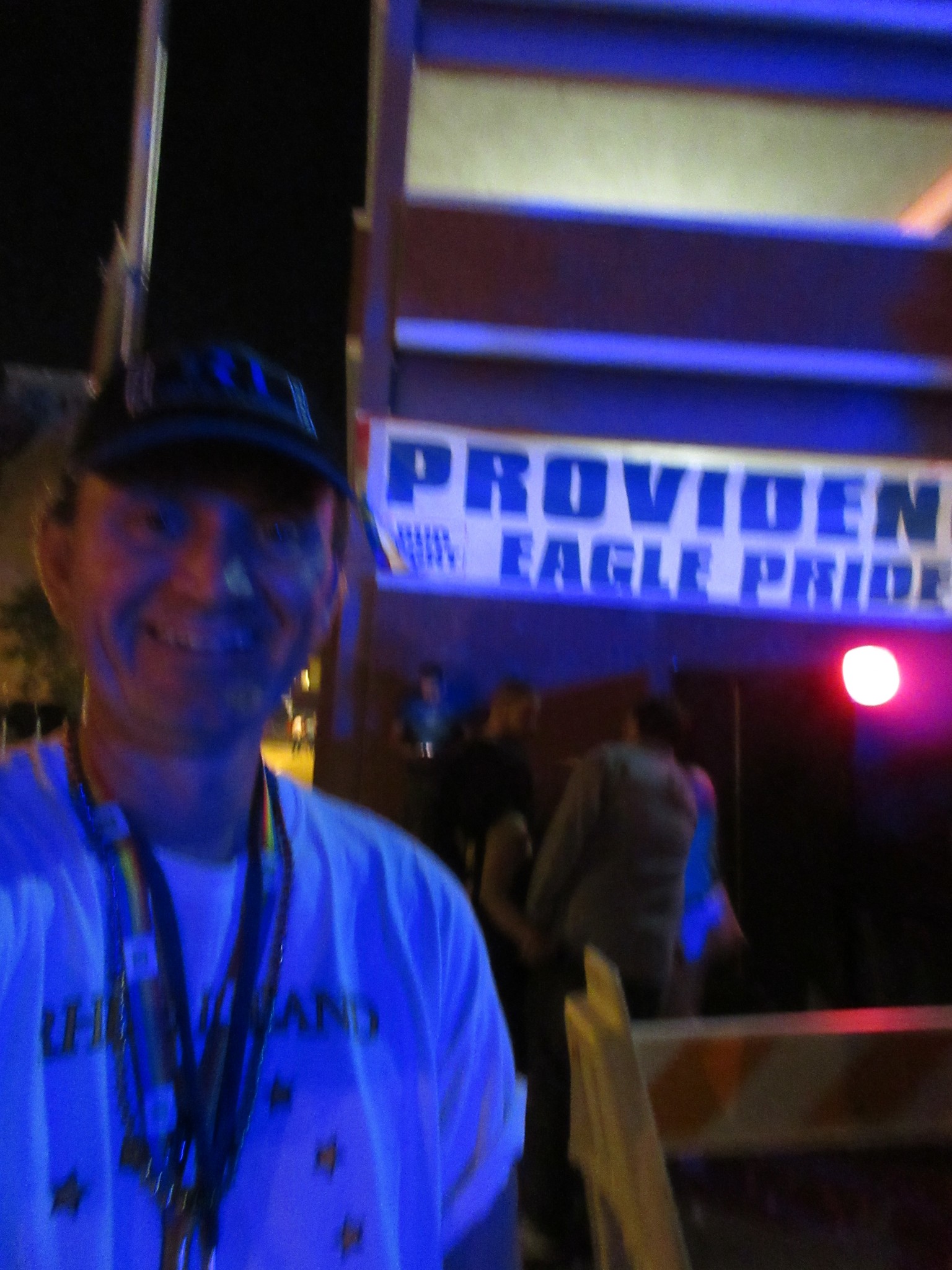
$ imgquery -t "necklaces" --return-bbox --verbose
[64,702,294,1269]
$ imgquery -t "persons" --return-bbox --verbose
[393,654,465,854]
[667,702,747,1027]
[0,336,531,1268]
[458,732,552,1103]
[428,672,543,892]
[524,686,700,1238]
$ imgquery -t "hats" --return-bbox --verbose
[72,342,363,512]
